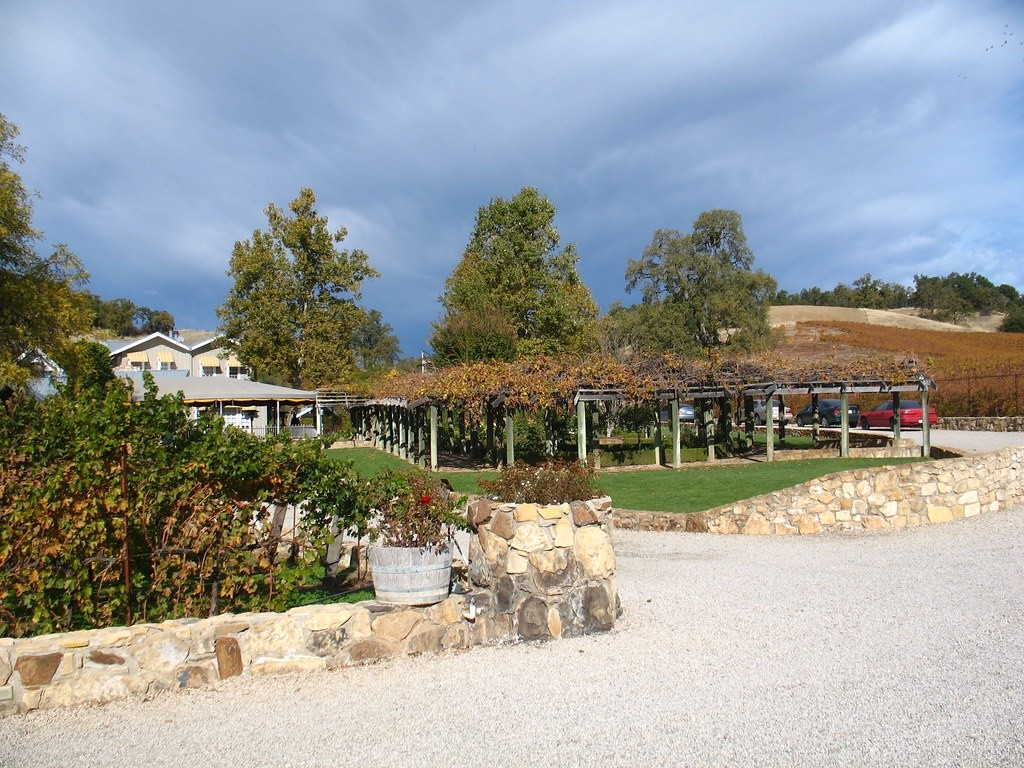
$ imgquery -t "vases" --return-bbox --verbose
[365,535,453,606]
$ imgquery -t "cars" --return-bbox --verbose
[859,399,937,430]
[733,399,793,426]
[659,403,694,423]
[797,399,859,428]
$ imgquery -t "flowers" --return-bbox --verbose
[357,466,469,555]
